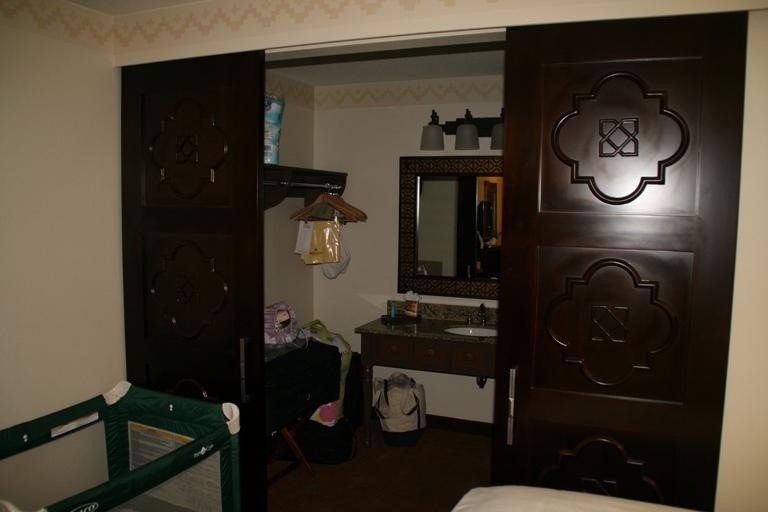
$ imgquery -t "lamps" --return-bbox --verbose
[420,108,504,152]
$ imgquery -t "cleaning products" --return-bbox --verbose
[403,291,419,317]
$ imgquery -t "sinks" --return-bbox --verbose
[444,324,498,337]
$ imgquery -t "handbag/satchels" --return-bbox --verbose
[264,301,299,344]
[373,371,427,432]
[300,320,352,427]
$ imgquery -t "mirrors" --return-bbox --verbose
[397,156,503,300]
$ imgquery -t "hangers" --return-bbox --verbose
[289,184,369,227]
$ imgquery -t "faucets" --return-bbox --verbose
[477,303,486,326]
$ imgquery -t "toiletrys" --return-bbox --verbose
[388,300,398,318]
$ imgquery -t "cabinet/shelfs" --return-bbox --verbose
[362,335,510,448]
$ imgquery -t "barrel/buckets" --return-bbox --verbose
[265,95,283,165]
